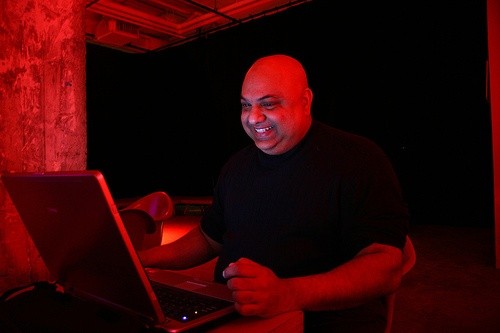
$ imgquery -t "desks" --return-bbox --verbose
[144,267,304,333]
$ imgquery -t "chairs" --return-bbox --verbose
[384,235,416,333]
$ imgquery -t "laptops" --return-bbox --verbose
[0,170,240,333]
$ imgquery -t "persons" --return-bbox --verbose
[137,55,408,333]
